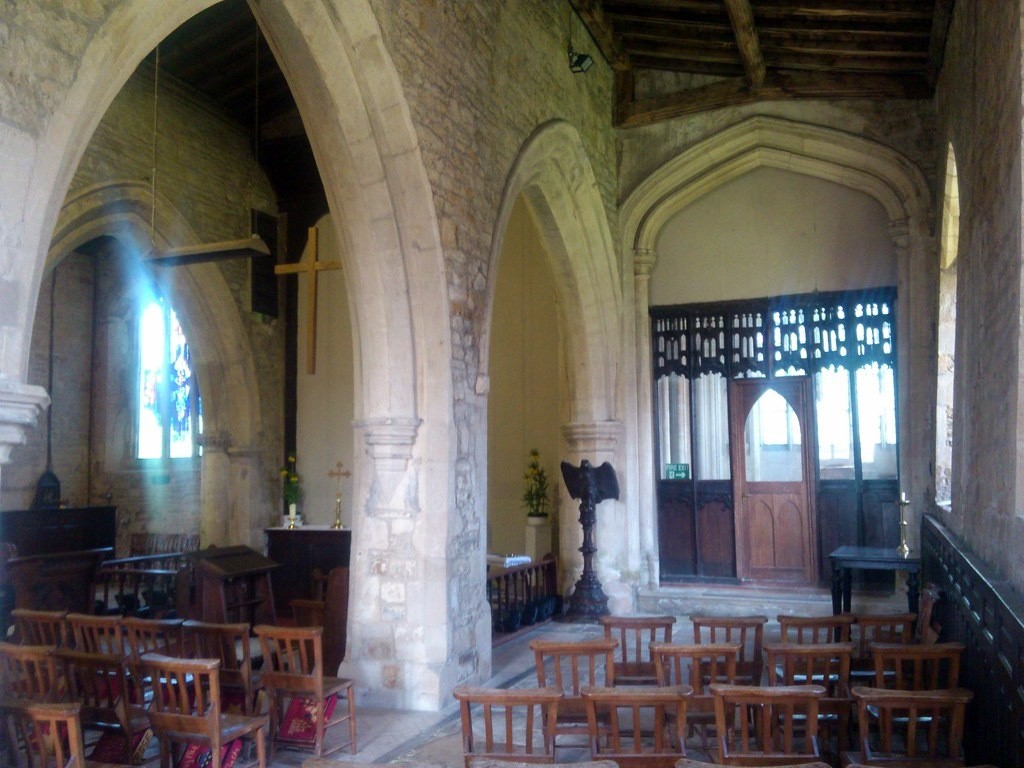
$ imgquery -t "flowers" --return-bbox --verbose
[523,446,551,513]
[279,456,302,512]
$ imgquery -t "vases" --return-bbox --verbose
[526,514,552,588]
[283,514,302,528]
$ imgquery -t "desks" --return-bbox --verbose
[830,547,920,643]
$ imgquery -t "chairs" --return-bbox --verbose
[852,613,916,664]
[600,615,677,683]
[0,535,356,768]
[839,686,973,768]
[529,638,618,754]
[850,643,965,746]
[649,642,741,754]
[707,684,826,763]
[455,684,564,768]
[688,611,767,686]
[776,611,853,682]
[757,643,855,750]
[583,685,693,768]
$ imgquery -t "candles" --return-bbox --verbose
[288,504,299,516]
[902,492,905,501]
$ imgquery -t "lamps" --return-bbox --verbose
[571,52,593,74]
[143,47,269,267]
